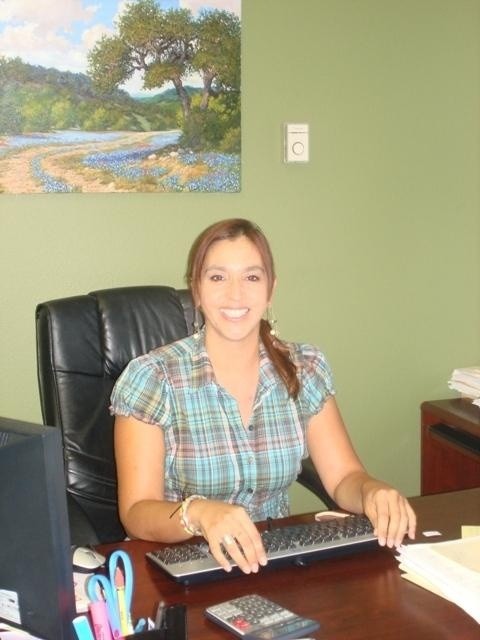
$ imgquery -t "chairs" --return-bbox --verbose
[34,285,204,549]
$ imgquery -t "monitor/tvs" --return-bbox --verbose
[0,415,76,640]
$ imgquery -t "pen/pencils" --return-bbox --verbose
[72,601,111,640]
[115,567,165,636]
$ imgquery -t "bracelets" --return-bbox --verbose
[180,494,207,536]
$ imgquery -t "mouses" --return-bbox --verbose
[70,543,106,574]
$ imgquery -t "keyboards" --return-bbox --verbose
[145,513,380,586]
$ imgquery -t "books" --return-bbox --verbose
[395,536,480,624]
[448,368,480,407]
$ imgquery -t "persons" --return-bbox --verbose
[109,219,417,574]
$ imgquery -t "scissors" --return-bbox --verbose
[87,550,134,640]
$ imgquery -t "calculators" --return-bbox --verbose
[203,594,320,639]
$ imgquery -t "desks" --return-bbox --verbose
[90,394,479,638]
